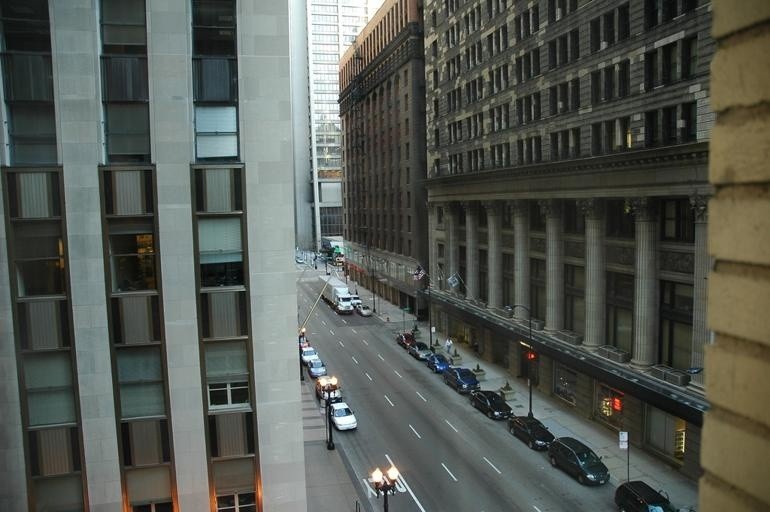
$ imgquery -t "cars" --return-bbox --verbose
[307,359,328,377]
[296,255,304,264]
[396,333,417,349]
[356,304,373,317]
[314,376,343,403]
[407,342,434,360]
[469,389,514,421]
[507,416,556,450]
[427,354,450,373]
[299,335,310,348]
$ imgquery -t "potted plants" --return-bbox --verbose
[450,348,462,365]
[411,325,421,338]
[430,338,443,354]
[499,380,515,400]
[472,363,486,380]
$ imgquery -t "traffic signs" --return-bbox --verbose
[619,431,628,441]
[619,441,628,449]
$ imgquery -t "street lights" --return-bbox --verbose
[374,466,398,512]
[360,252,376,315]
[298,327,306,380]
[317,375,341,450]
[504,303,533,418]
[422,281,432,348]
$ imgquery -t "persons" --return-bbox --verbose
[446,338,453,354]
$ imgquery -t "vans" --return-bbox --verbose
[547,436,610,486]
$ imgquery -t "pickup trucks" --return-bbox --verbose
[442,365,481,393]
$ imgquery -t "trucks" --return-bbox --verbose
[319,275,354,315]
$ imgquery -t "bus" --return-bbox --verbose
[328,402,358,432]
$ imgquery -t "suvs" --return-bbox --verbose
[350,293,363,308]
[301,347,320,366]
[614,479,676,512]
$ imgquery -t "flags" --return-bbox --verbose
[414,266,425,280]
[447,274,462,289]
